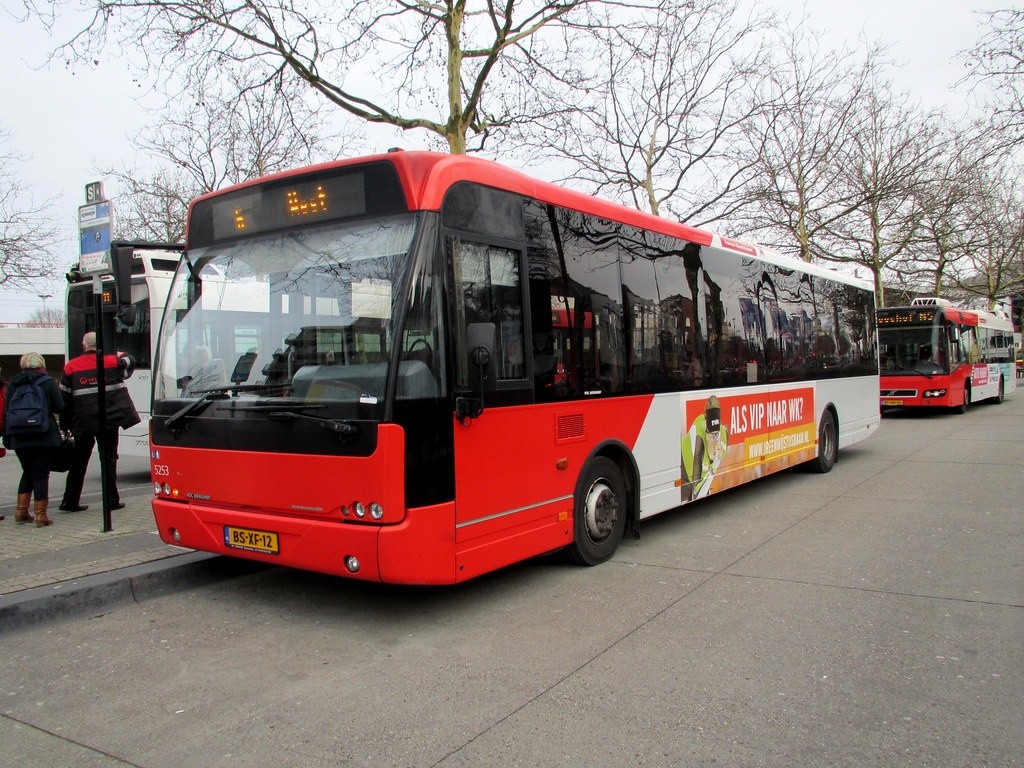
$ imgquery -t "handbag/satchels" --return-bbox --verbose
[50,435,74,473]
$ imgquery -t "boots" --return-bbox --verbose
[34,498,53,528]
[15,492,34,524]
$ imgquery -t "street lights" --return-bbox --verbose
[40,294,52,309]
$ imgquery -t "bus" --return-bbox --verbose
[876,298,1017,415]
[68,249,431,478]
[148,147,880,587]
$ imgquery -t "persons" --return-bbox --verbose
[682,396,729,498]
[60,332,136,512]
[179,346,210,386]
[0,353,65,526]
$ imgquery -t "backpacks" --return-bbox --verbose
[5,374,54,435]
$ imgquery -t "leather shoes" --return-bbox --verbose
[111,503,125,510]
[59,501,89,511]
